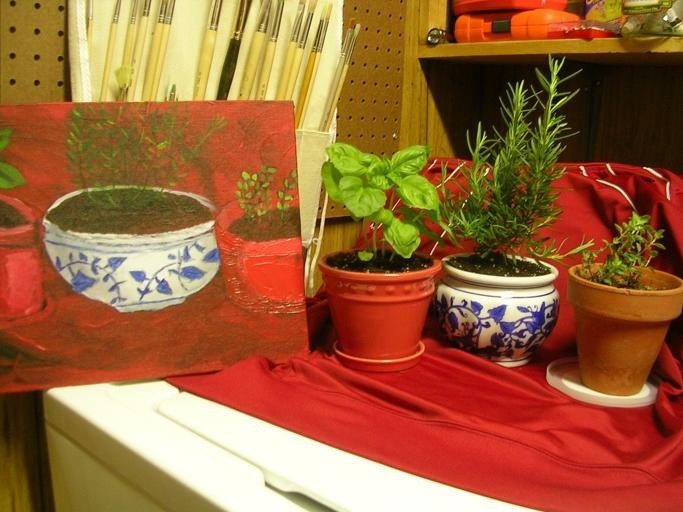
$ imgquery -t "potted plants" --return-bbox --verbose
[439,52,593,369]
[40,83,220,317]
[0,128,45,323]
[322,143,444,360]
[223,167,304,316]
[566,208,683,395]
